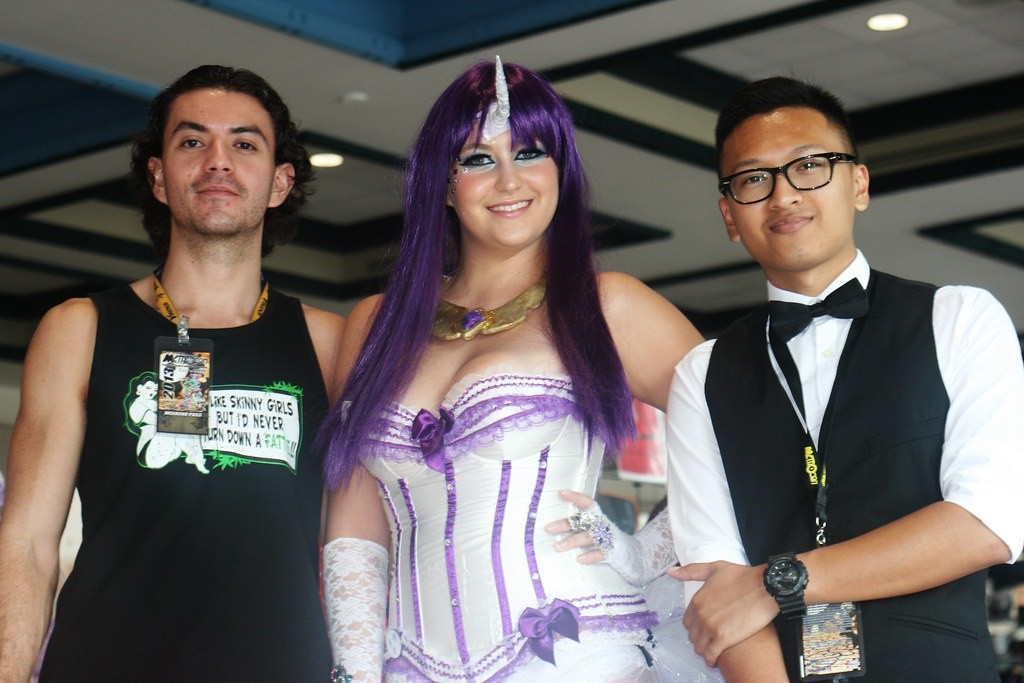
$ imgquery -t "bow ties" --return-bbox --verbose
[767,277,868,346]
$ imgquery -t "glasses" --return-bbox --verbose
[718,152,858,205]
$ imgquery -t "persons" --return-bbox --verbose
[660,76,1024,683]
[0,65,348,683]
[319,56,707,683]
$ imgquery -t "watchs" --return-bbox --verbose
[763,551,809,622]
